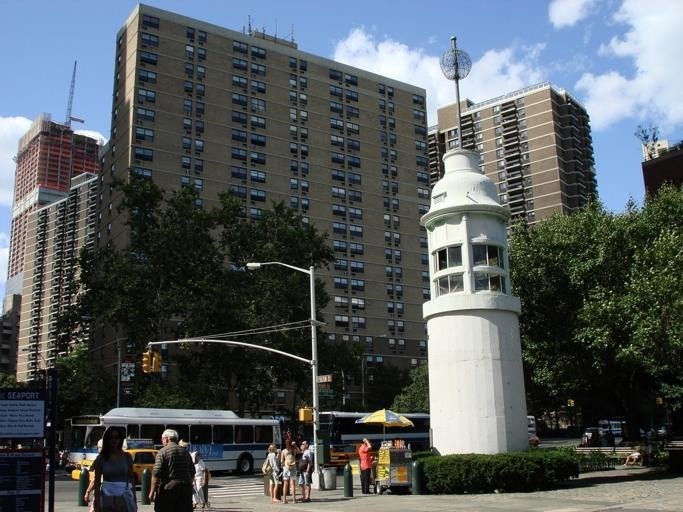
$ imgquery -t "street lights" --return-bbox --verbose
[99,495,129,511]
[298,458,308,472]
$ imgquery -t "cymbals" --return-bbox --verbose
[64,59,85,127]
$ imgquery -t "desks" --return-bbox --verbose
[22,348,48,389]
[246,261,319,470]
[81,315,121,408]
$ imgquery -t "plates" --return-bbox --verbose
[376,446,412,487]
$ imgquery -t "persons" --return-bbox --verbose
[647,426,667,439]
[358,437,374,494]
[589,430,615,447]
[261,439,314,504]
[84,426,211,512]
[624,441,653,467]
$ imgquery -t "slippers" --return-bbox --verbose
[285,459,296,470]
[262,459,272,475]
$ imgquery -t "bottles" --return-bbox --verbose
[190,450,198,463]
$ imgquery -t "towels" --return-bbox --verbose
[320,467,337,490]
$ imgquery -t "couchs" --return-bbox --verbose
[656,397,663,404]
[567,399,576,407]
[142,350,162,373]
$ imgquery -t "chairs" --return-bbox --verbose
[296,496,312,502]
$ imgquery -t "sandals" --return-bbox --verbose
[599,422,625,436]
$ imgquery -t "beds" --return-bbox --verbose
[355,407,414,443]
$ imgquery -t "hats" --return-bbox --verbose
[641,427,675,440]
[72,449,160,483]
[529,433,540,446]
[584,427,603,440]
